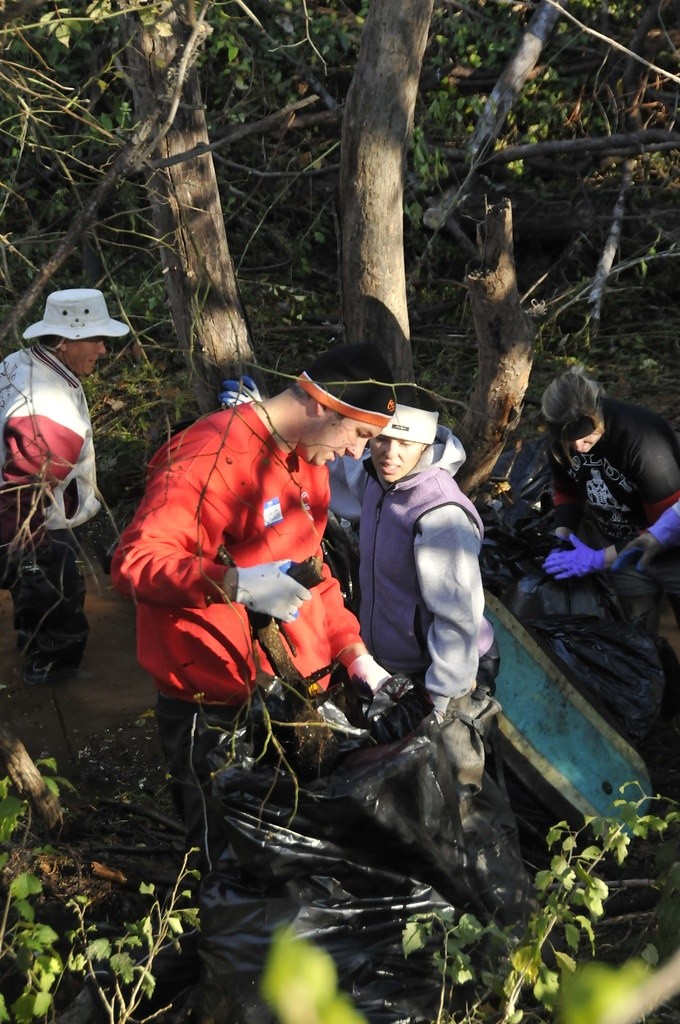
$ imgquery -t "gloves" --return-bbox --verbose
[610,532,663,571]
[218,375,264,408]
[543,532,606,579]
[235,558,312,624]
[347,655,414,721]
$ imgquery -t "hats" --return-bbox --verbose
[296,343,398,429]
[22,288,131,340]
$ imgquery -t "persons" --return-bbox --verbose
[539,372,680,579]
[216,376,500,717]
[110,344,398,835]
[0,290,129,678]
